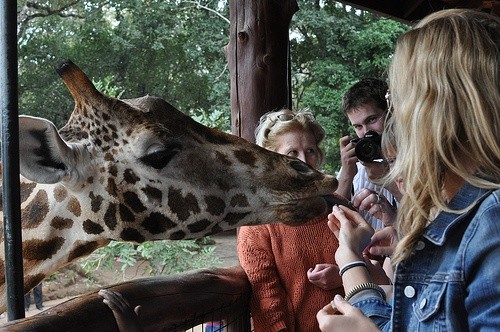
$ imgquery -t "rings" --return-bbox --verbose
[376,193,381,203]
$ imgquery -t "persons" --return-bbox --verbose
[316,8,500,332]
[25,282,43,310]
[236,108,370,332]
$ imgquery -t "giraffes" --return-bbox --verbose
[0,55,342,316]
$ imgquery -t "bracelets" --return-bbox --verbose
[344,282,386,301]
[339,261,366,276]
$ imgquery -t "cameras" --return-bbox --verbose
[347,130,381,163]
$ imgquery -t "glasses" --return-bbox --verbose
[385,90,394,114]
[270,113,315,128]
